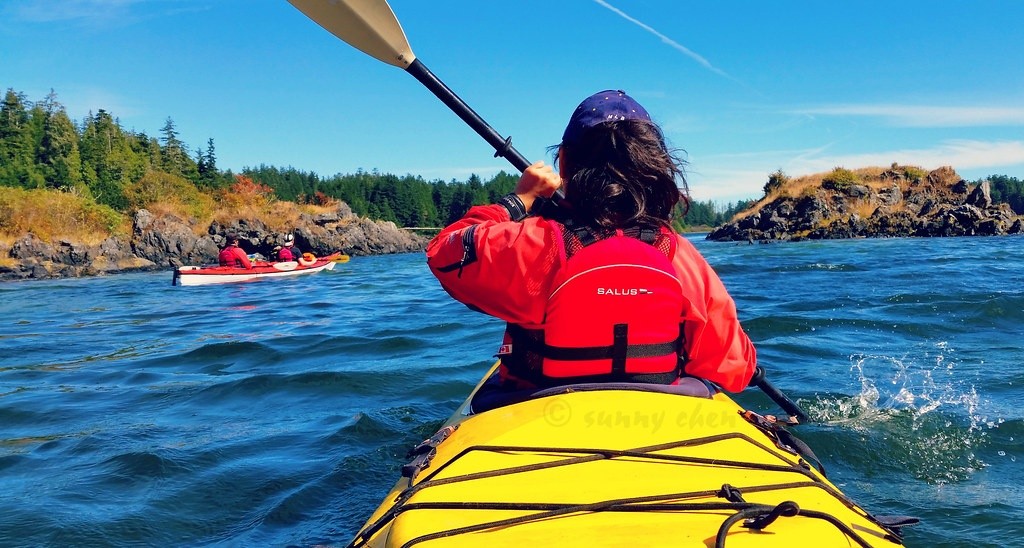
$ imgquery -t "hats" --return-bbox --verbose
[562,90,667,171]
[284,233,295,246]
[225,233,237,242]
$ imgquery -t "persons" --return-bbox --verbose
[425,90,757,392]
[276,235,303,262]
[219,233,252,270]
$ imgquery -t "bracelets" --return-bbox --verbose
[497,192,528,222]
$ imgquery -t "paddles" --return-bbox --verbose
[247,254,350,264]
[288,0,814,425]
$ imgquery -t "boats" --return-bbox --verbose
[179,252,341,287]
